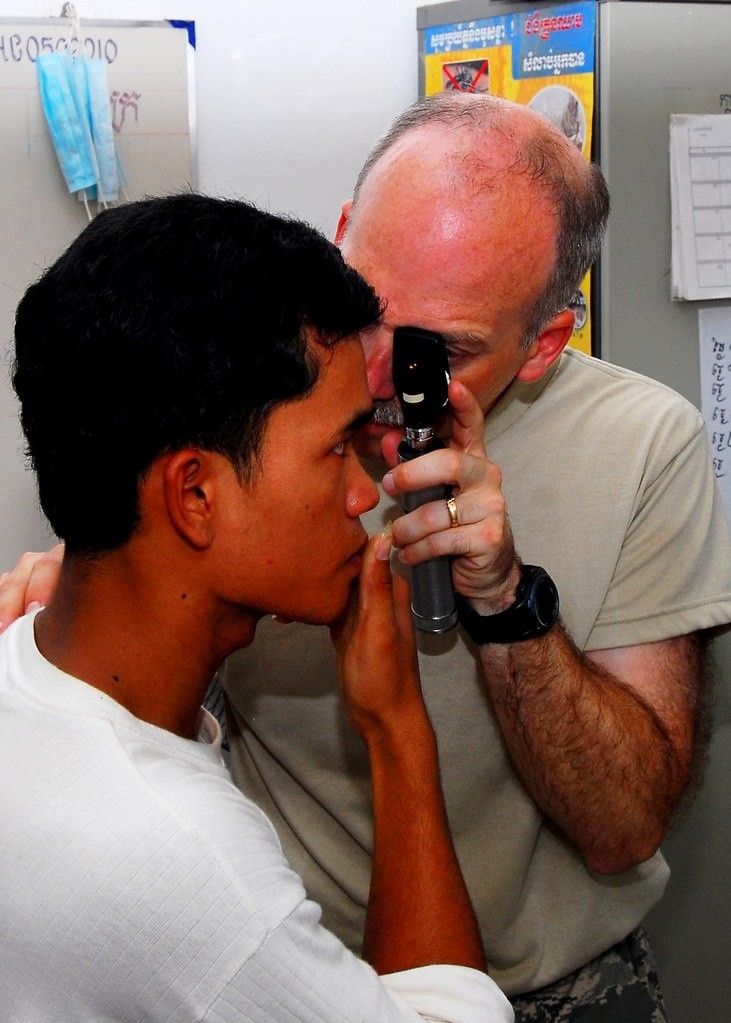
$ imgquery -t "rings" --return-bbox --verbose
[446,495,459,527]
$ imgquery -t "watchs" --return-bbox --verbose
[454,564,560,644]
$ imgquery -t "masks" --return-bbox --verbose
[40,49,132,222]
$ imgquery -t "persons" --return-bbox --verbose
[0,89,730,1023]
[0,187,520,1022]
[561,94,583,153]
